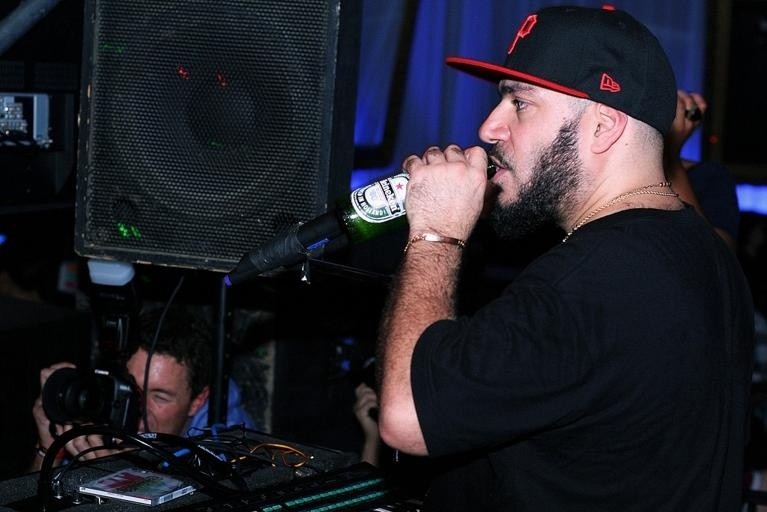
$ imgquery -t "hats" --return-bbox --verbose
[445,5,678,138]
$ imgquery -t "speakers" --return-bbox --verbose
[72,1,340,280]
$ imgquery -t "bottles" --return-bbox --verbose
[336,158,497,245]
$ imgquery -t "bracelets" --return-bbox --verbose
[33,441,66,460]
[400,229,467,258]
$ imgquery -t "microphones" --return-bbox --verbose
[224,208,338,290]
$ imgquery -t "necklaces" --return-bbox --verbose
[556,179,679,244]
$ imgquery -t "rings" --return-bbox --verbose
[684,107,705,124]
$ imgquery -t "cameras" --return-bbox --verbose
[42,367,143,433]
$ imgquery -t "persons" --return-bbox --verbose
[661,88,740,253]
[376,2,752,511]
[351,380,379,469]
[0,231,74,338]
[15,320,263,478]
